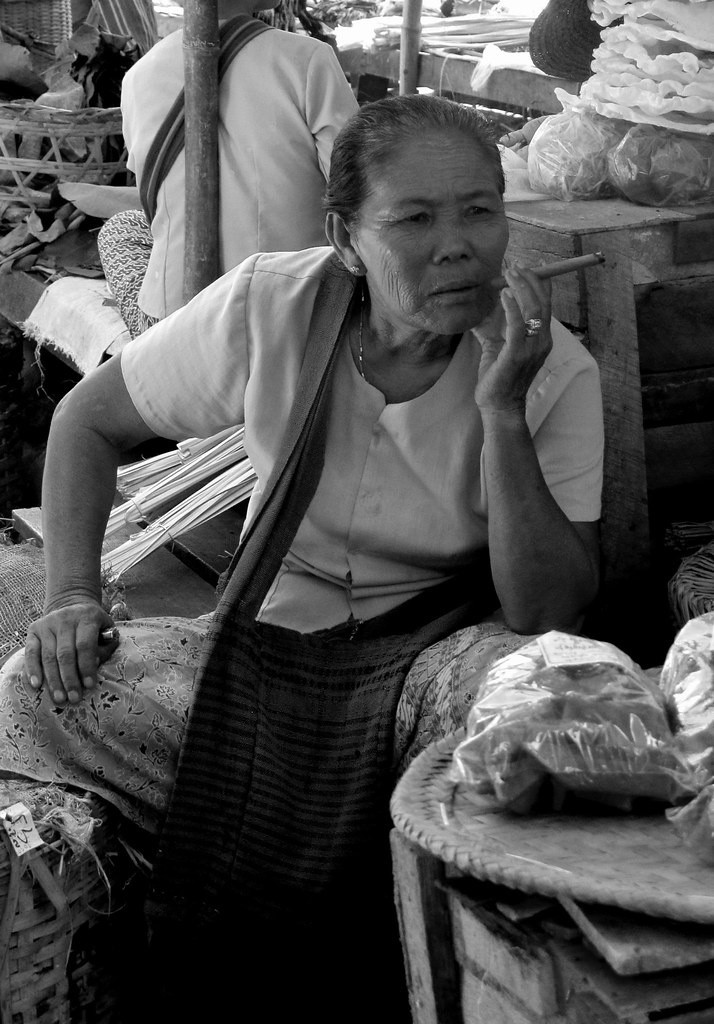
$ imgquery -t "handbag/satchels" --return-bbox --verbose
[528,87,621,201]
[609,123,711,207]
[144,621,468,1024]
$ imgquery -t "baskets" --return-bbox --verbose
[0,0,73,62]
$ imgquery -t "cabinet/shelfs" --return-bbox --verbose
[502,199,714,622]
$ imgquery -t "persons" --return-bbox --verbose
[0,1,605,1024]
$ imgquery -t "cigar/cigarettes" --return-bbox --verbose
[490,251,603,290]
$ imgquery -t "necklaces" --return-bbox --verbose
[359,307,366,379]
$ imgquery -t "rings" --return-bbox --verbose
[523,318,543,329]
[525,331,538,337]
